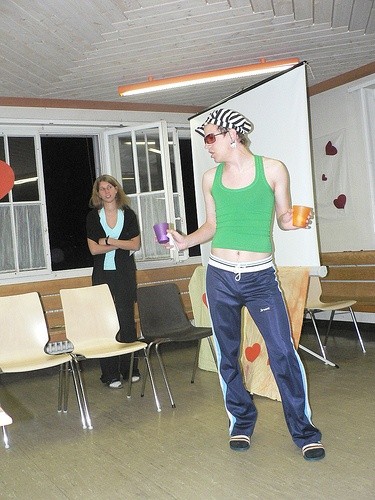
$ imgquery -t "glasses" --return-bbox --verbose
[203,131,228,144]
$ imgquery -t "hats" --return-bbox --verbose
[193,108,251,136]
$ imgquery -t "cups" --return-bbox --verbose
[153,223,169,244]
[293,205,311,228]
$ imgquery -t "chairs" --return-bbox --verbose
[137,284,218,408]
[60,283,161,430]
[305,276,367,364]
[0,292,87,449]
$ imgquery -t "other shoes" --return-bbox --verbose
[103,377,123,388]
[122,369,140,384]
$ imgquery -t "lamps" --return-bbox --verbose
[118,57,300,97]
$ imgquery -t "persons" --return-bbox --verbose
[155,108,325,461]
[84,174,141,388]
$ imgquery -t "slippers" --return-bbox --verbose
[300,440,325,461]
[229,434,250,451]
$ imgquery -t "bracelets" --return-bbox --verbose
[105,236,110,245]
